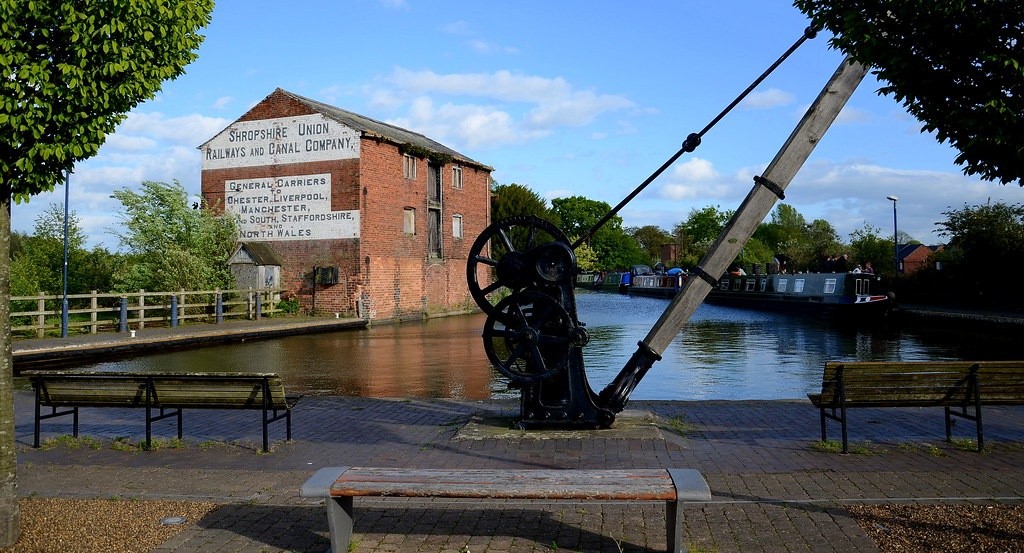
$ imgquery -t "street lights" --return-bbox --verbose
[886,194,899,306]
[671,234,677,267]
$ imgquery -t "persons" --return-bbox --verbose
[730,264,747,276]
[777,267,796,276]
[852,262,873,273]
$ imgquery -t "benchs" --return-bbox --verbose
[807,360,1024,455]
[299,466,712,553]
[20,368,304,454]
